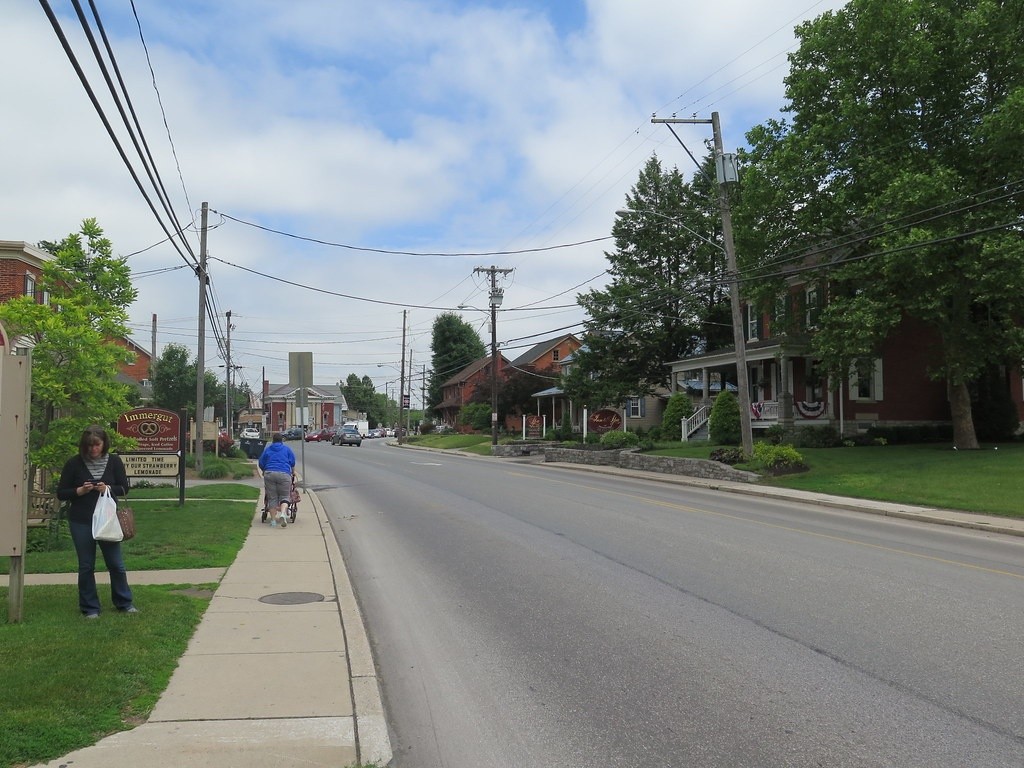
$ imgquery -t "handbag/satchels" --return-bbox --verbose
[92,486,124,542]
[111,486,136,540]
[290,489,301,503]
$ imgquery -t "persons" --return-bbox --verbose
[56,428,137,619]
[258,432,296,527]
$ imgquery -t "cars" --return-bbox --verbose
[331,428,362,447]
[240,427,260,440]
[394,428,406,438]
[379,428,387,438]
[392,429,396,438]
[386,427,392,438]
[280,428,306,440]
[305,428,337,442]
[369,429,382,438]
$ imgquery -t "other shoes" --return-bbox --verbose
[269,521,277,527]
[128,608,138,613]
[87,614,98,619]
[280,515,287,527]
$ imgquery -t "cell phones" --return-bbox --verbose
[91,482,98,485]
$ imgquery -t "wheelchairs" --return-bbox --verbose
[260,472,301,523]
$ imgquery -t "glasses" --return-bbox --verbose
[87,431,101,434]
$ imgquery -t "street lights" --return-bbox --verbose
[615,208,755,460]
[457,304,498,446]
[377,363,404,445]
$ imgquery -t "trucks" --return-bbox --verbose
[342,420,370,438]
